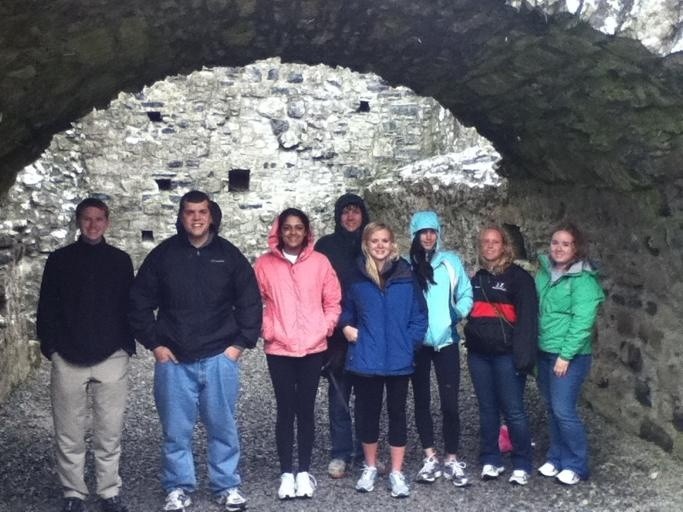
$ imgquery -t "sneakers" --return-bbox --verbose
[95,497,128,512]
[160,488,193,512]
[355,464,378,493]
[538,462,558,477]
[414,456,442,483]
[508,469,530,485]
[294,472,316,498]
[442,458,469,488]
[352,459,387,475]
[386,471,409,497]
[215,490,248,511]
[63,496,90,512]
[554,468,581,485]
[480,464,504,480]
[327,459,348,478]
[277,473,296,500]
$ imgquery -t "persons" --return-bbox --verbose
[311,192,371,479]
[536,222,605,485]
[252,207,342,500]
[38,199,134,512]
[403,211,474,487]
[465,225,539,486]
[129,191,263,512]
[340,221,429,495]
